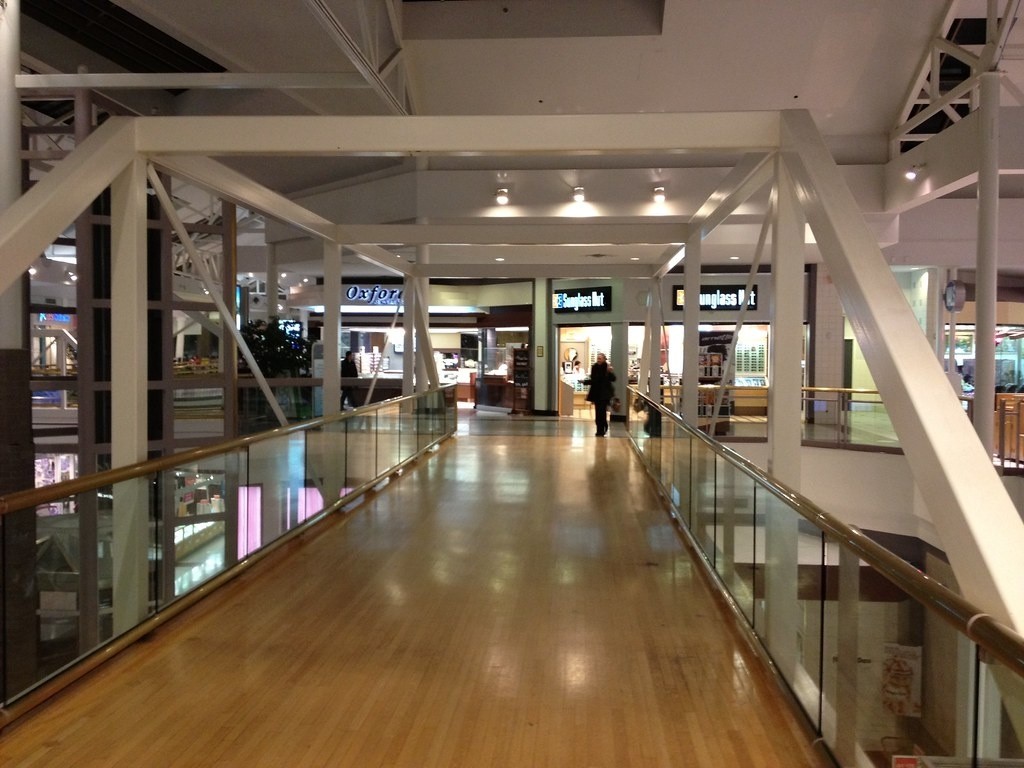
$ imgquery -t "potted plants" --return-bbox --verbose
[238,321,311,420]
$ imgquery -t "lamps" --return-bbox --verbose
[654,190,665,202]
[573,189,585,203]
[496,191,509,205]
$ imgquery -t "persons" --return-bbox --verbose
[573,361,585,376]
[340,351,358,410]
[578,353,617,438]
[962,374,975,409]
